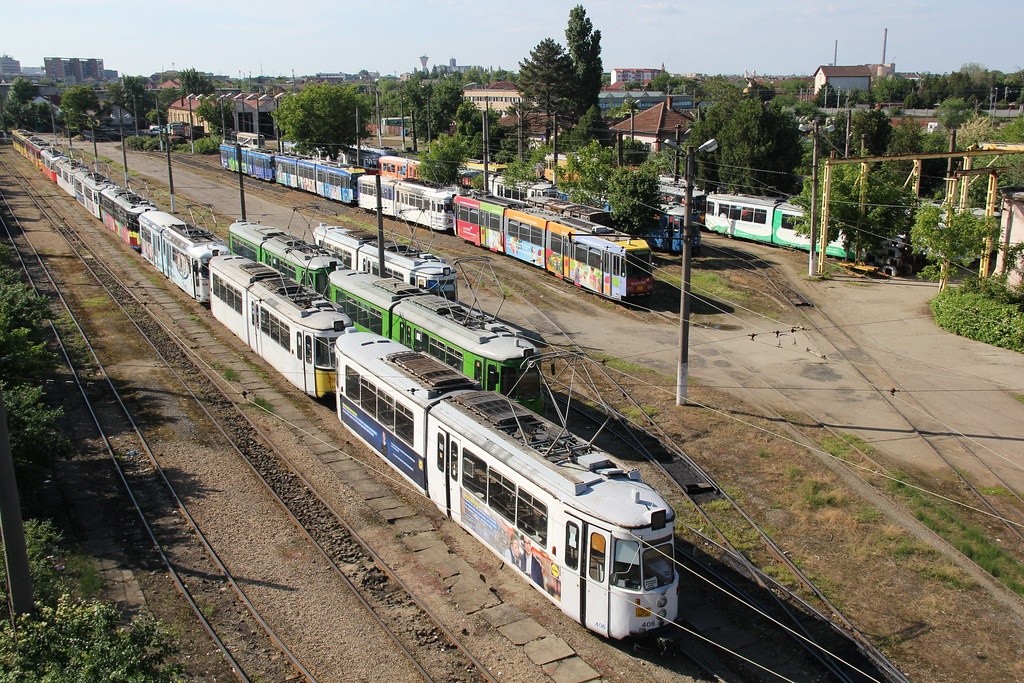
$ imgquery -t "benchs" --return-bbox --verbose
[474,483,502,497]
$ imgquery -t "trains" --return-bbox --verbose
[10,124,683,641]
[224,217,547,415]
[312,220,460,304]
[218,139,656,305]
[237,117,917,277]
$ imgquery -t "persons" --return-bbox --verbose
[381,432,388,458]
[529,247,536,264]
[573,262,597,290]
[708,207,763,222]
[502,533,545,590]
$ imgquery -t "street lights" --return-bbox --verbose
[797,119,837,277]
[663,137,720,407]
[150,123,176,215]
[110,108,131,189]
[222,136,253,223]
[58,107,74,158]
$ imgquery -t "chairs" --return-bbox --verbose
[517,515,536,534]
[505,508,515,522]
[490,494,507,511]
